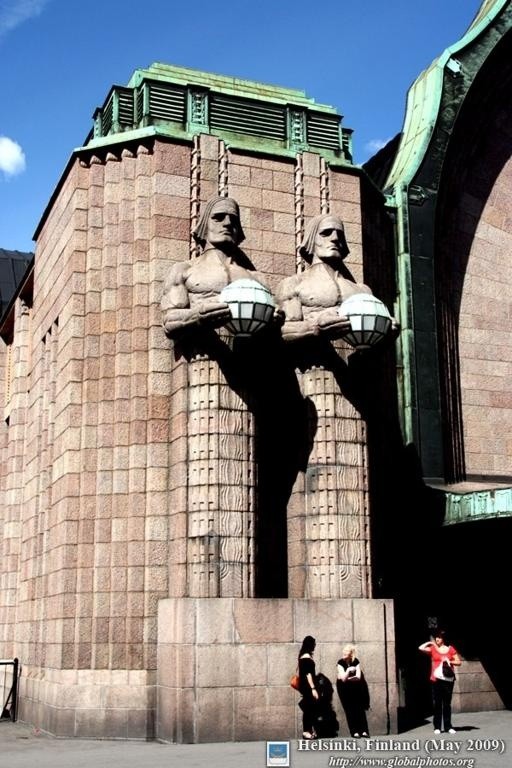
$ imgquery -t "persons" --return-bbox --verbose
[417,631,462,735]
[274,211,404,346]
[335,645,371,739]
[159,194,289,341]
[297,634,321,740]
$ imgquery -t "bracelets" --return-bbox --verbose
[311,687,316,690]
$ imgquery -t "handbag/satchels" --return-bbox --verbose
[289,675,300,692]
[433,661,456,683]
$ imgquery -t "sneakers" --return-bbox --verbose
[302,728,457,739]
